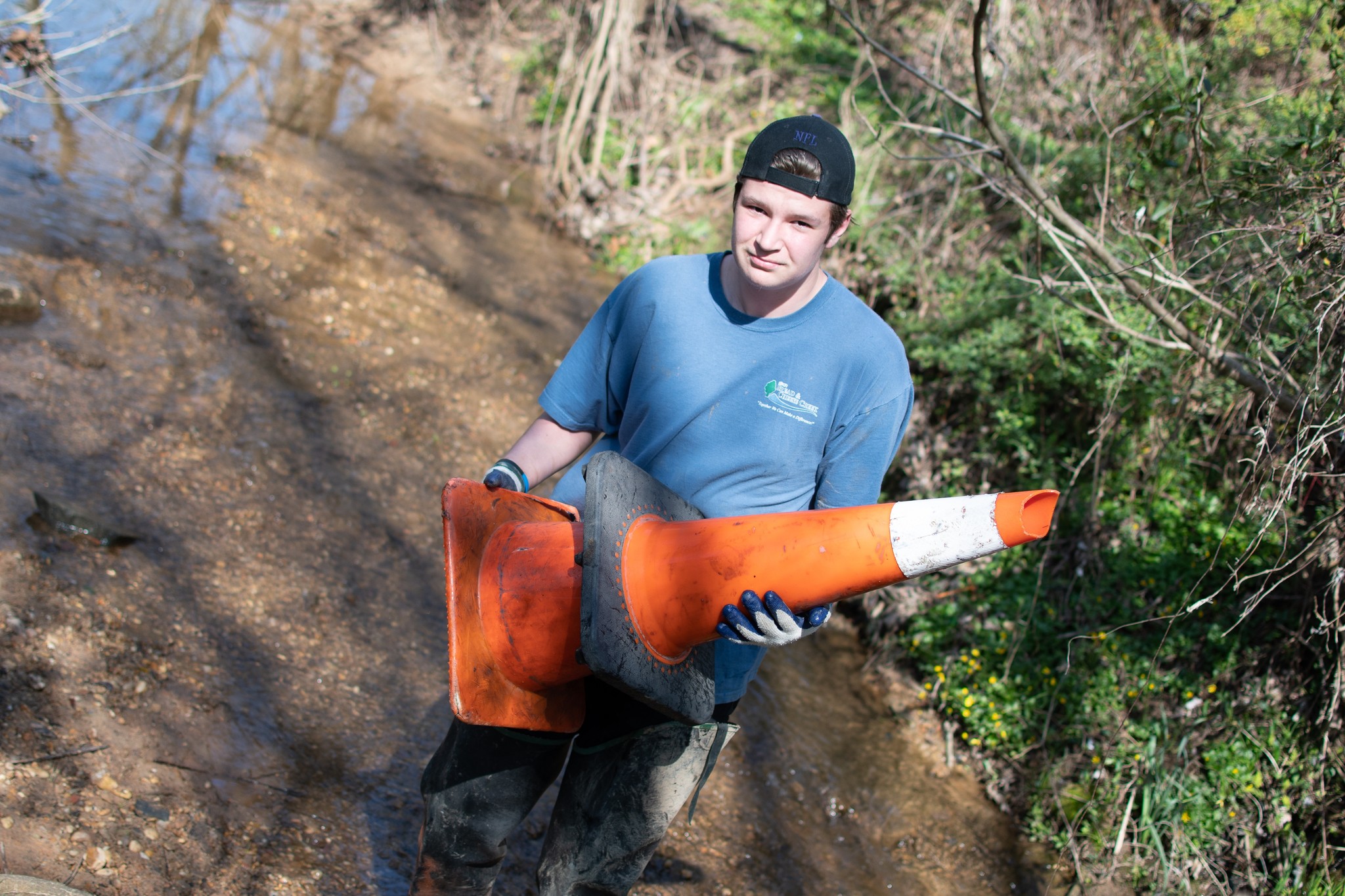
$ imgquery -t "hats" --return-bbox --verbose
[741,115,856,205]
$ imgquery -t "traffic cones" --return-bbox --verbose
[443,449,1061,734]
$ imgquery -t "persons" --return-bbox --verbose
[408,115,913,896]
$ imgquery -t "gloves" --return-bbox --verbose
[478,459,529,494]
[717,589,831,647]
